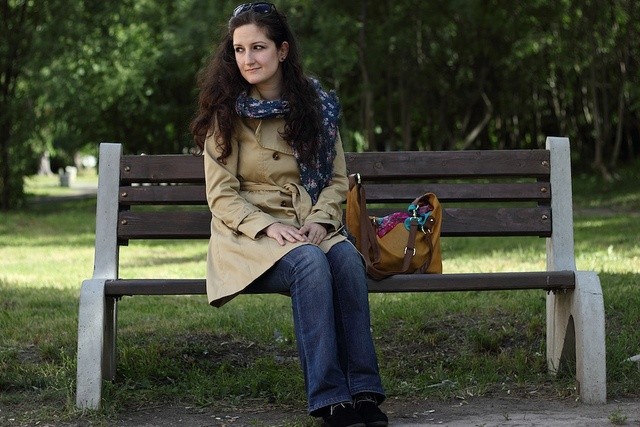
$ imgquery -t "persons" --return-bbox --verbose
[190,1,389,426]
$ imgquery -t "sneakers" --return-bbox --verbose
[321,401,366,427]
[355,399,388,427]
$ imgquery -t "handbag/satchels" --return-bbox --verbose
[346,171,443,281]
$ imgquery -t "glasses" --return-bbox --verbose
[232,2,279,18]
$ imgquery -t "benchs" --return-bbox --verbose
[75,135,607,414]
